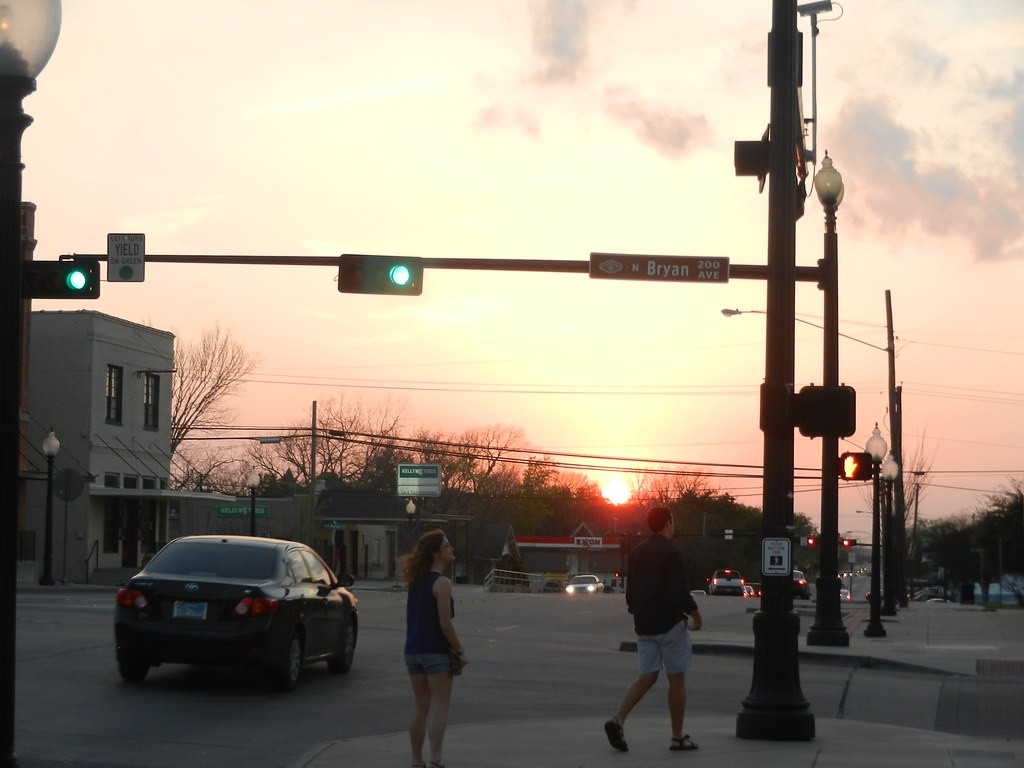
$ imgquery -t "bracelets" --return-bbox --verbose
[449,648,465,656]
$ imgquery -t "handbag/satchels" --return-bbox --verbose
[450,648,462,675]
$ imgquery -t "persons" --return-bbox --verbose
[402,530,468,768]
[603,505,701,752]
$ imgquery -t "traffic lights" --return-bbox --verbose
[19,261,101,300]
[841,538,858,547]
[797,384,857,437]
[864,425,886,638]
[338,253,423,296]
[807,537,821,546]
[840,452,873,481]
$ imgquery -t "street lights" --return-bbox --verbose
[38,427,60,585]
[722,309,909,609]
[806,148,852,648]
[0,1,64,768]
[881,450,898,616]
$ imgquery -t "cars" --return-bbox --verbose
[711,570,745,596]
[793,570,810,600]
[907,586,952,604]
[566,575,606,593]
[745,586,754,595]
[745,583,759,596]
[114,535,359,690]
[840,589,851,603]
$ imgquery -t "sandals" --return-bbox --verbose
[605,717,627,751]
[669,735,698,751]
